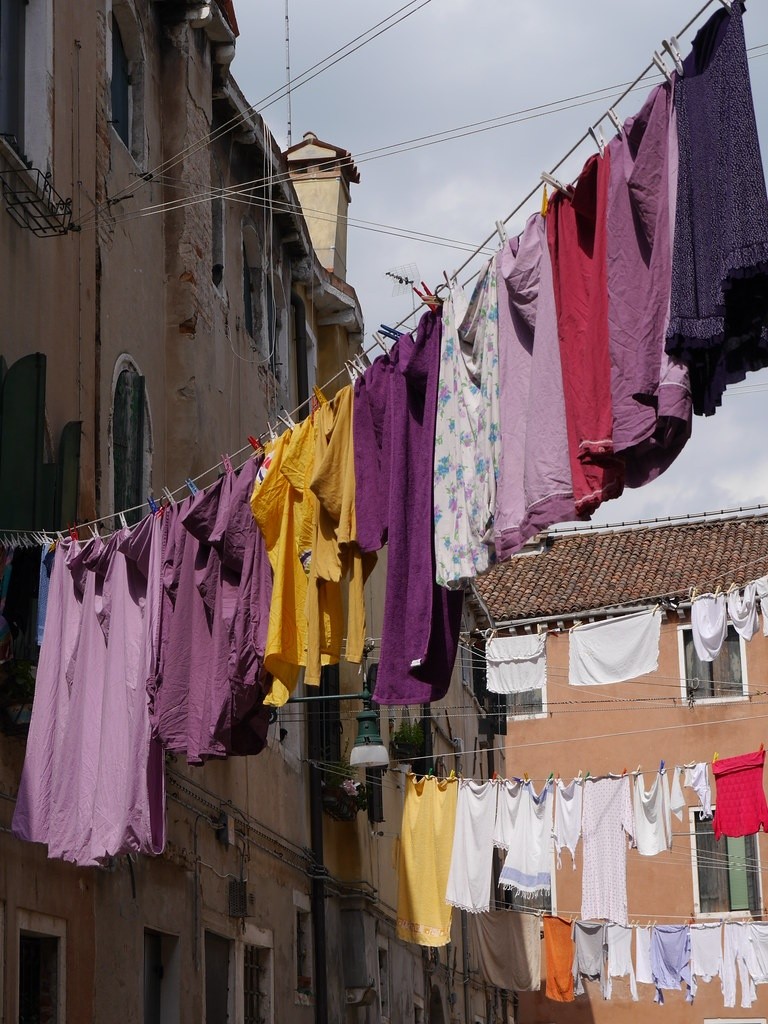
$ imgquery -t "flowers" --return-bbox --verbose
[325,755,368,810]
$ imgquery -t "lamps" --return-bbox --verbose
[288,674,391,768]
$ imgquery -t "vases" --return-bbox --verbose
[321,786,357,823]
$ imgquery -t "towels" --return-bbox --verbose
[568,609,662,686]
[371,311,464,705]
[543,915,575,1002]
[395,773,458,947]
[467,909,541,991]
[485,634,546,694]
[353,355,391,551]
